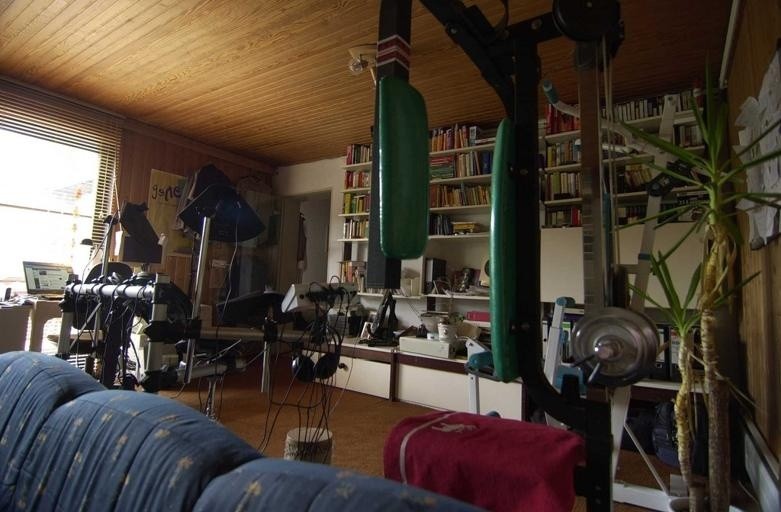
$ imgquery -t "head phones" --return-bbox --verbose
[292,322,343,383]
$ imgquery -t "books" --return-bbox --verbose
[425,258,447,283]
[429,122,493,235]
[538,90,703,229]
[342,143,372,239]
[341,259,367,292]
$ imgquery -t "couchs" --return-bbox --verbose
[0,352,484,512]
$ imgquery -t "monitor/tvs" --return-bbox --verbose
[22,261,74,300]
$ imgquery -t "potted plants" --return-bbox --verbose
[616,56,780,512]
[438,312,466,343]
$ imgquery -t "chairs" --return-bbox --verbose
[30,301,64,353]
[0,307,30,353]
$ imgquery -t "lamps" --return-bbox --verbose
[348,43,385,73]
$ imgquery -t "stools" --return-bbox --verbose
[284,426,332,465]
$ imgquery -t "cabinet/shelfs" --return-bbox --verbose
[338,107,706,243]
[297,338,394,400]
[393,353,525,422]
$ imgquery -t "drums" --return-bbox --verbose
[122,272,193,344]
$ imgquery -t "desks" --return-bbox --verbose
[0,298,63,308]
